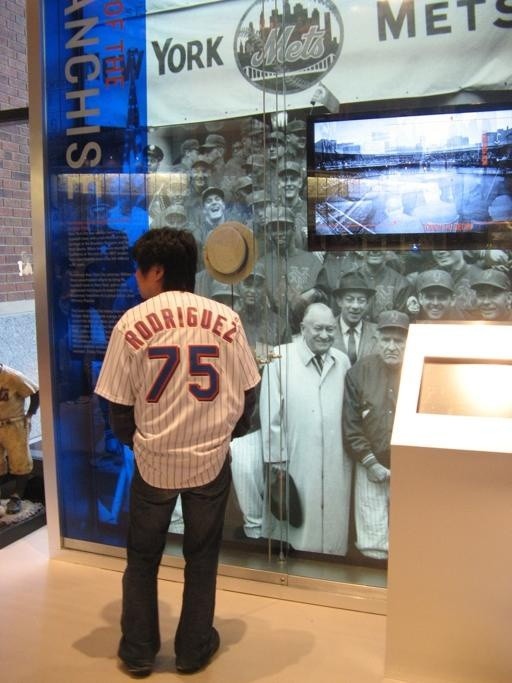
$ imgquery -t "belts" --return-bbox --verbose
[0,415,26,426]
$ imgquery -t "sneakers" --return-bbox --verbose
[331,270,508,329]
[6,496,21,514]
[177,626,220,673]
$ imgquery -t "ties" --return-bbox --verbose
[347,328,357,364]
[315,355,324,371]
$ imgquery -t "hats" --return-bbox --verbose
[268,471,303,528]
[148,122,303,299]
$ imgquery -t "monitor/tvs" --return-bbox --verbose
[307,102,512,251]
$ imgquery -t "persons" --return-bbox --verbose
[0,362,39,516]
[49,106,512,565]
[95,228,262,679]
